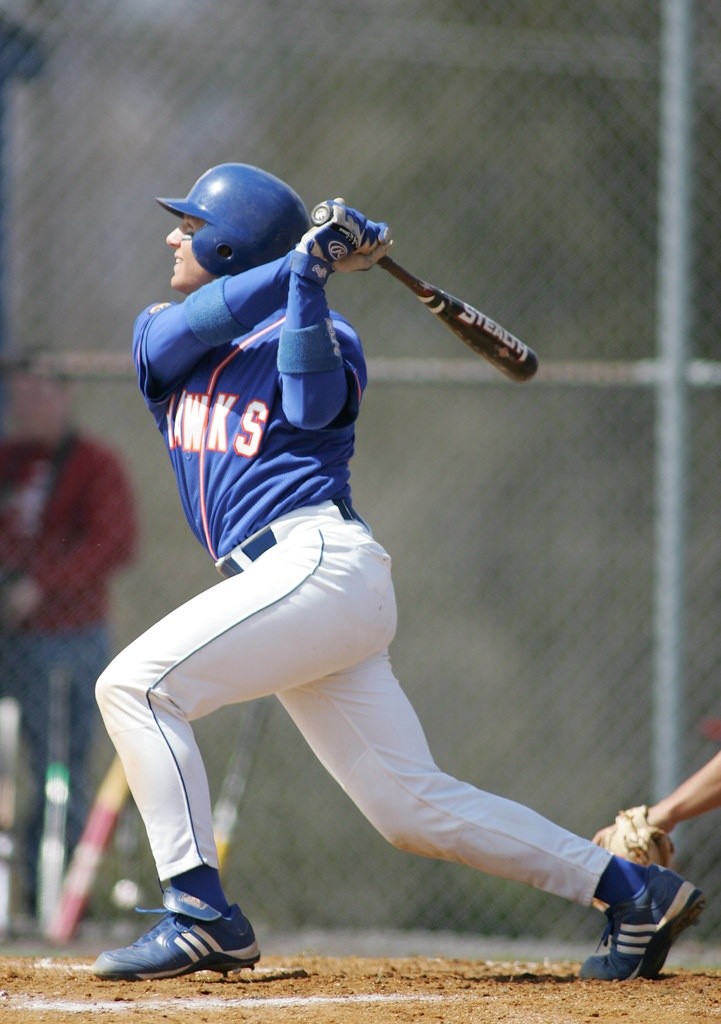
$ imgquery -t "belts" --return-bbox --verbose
[218,497,356,579]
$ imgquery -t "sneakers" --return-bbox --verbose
[92,877,260,981]
[580,864,706,982]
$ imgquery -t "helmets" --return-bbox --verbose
[156,163,310,276]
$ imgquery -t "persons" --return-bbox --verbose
[586,747,721,916]
[92,161,709,985]
[0,344,137,927]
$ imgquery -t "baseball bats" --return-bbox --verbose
[310,200,538,382]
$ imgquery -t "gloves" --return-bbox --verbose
[295,198,393,272]
